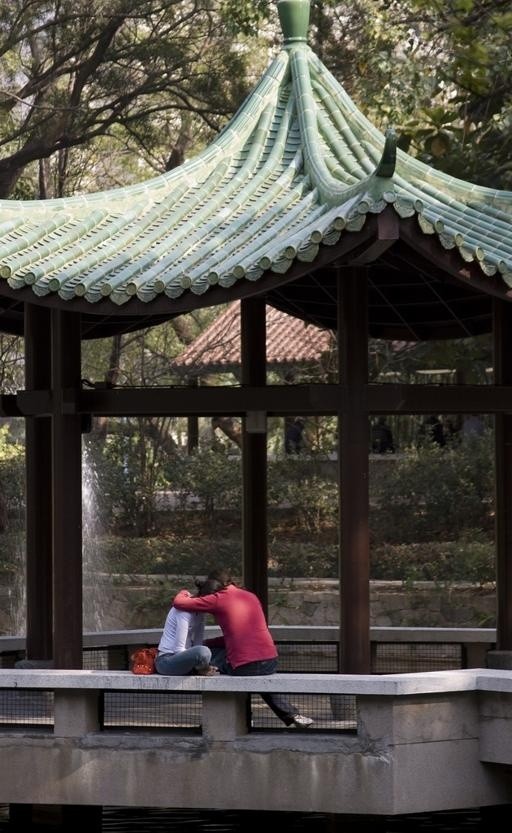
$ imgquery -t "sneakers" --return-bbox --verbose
[294,715,313,728]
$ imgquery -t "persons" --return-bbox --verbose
[154,578,223,676]
[173,569,314,729]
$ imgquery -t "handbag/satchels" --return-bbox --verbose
[133,648,157,673]
